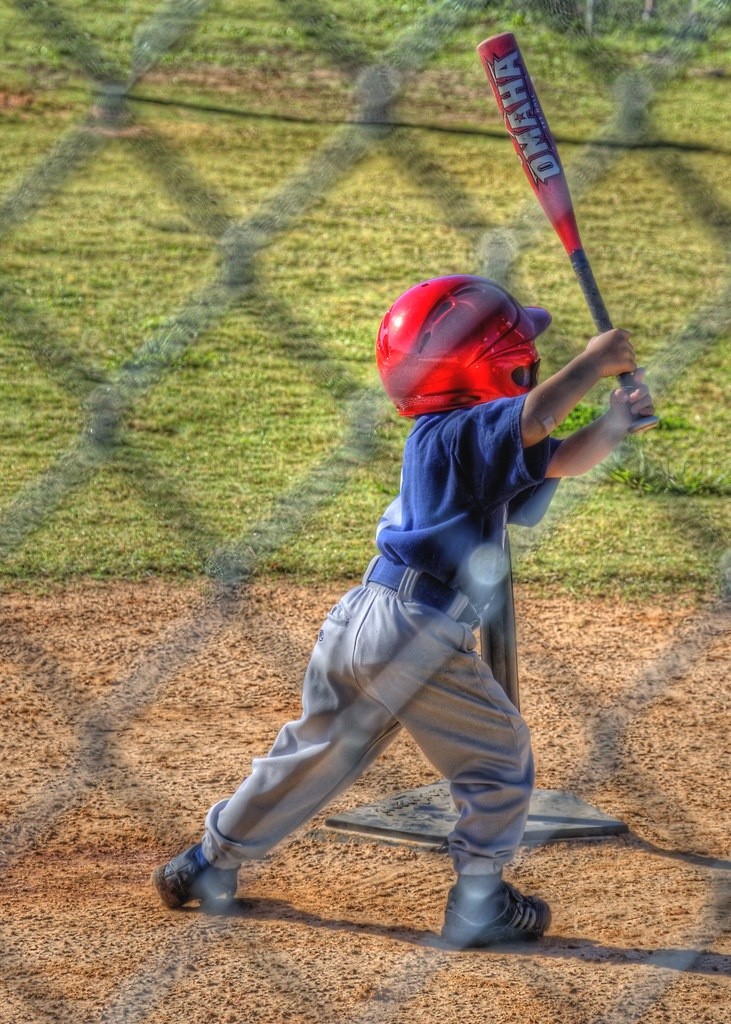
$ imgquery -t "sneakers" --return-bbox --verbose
[152,845,242,909]
[441,880,552,946]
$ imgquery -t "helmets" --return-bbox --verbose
[376,276,553,416]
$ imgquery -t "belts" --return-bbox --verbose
[370,557,483,633]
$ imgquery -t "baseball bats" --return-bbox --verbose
[475,30,662,436]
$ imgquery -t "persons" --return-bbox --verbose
[153,274,657,947]
